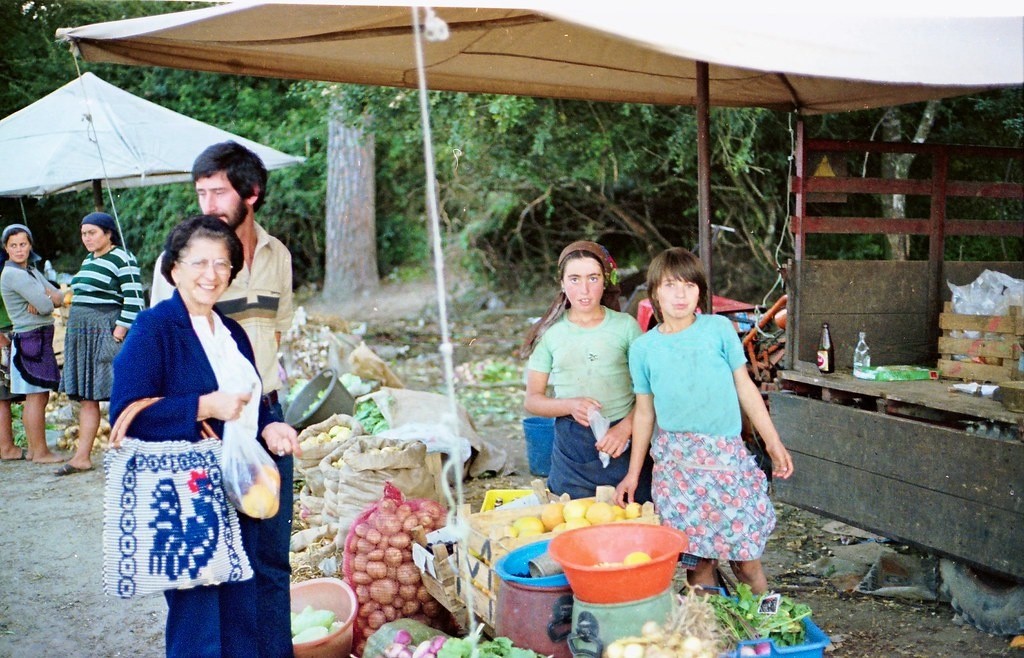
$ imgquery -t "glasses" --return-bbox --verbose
[175,257,234,275]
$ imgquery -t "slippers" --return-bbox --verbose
[2,449,29,461]
[53,463,96,476]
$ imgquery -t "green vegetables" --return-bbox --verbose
[11,403,62,448]
[353,397,394,434]
[437,634,554,658]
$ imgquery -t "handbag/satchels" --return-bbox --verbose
[102,396,255,596]
[214,382,281,518]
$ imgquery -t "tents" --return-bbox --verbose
[0,72,300,311]
[58,4,1024,658]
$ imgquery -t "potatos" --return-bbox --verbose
[348,499,448,655]
[58,418,111,453]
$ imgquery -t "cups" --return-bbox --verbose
[527,552,563,578]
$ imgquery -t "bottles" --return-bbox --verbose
[854,332,870,368]
[817,323,835,374]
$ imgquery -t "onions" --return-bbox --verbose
[602,621,719,658]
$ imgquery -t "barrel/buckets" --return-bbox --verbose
[495,580,574,658]
[567,581,673,658]
[523,417,556,477]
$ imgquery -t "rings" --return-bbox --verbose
[278,451,285,456]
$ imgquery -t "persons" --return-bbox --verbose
[54,212,144,477]
[524,241,654,505]
[109,214,302,658]
[1,224,71,463]
[151,142,294,658]
[0,246,28,460]
[609,248,793,596]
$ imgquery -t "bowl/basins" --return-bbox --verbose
[998,381,1024,413]
[548,523,689,604]
[493,540,569,587]
[285,368,355,433]
[290,577,358,658]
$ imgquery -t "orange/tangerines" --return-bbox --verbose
[466,497,651,595]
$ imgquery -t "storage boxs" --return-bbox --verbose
[936,301,1024,384]
[703,586,830,658]
[410,476,661,627]
